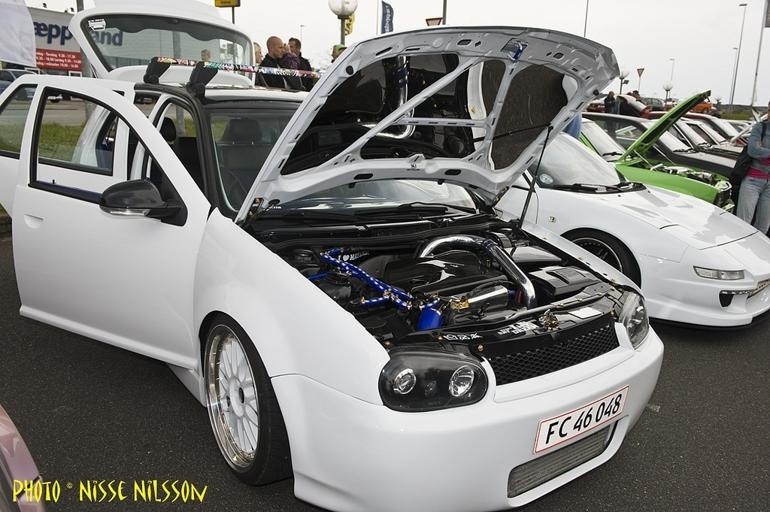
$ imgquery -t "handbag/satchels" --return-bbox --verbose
[729,144,754,209]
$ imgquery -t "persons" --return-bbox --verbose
[331,45,346,62]
[736,101,770,235]
[200,49,211,62]
[604,91,645,131]
[254,37,314,93]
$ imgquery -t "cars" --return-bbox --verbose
[0,0,674,512]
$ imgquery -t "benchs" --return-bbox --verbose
[176,136,217,188]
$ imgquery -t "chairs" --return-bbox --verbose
[216,117,277,204]
[143,114,185,203]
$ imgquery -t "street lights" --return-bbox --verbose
[326,0,359,45]
[730,2,748,106]
